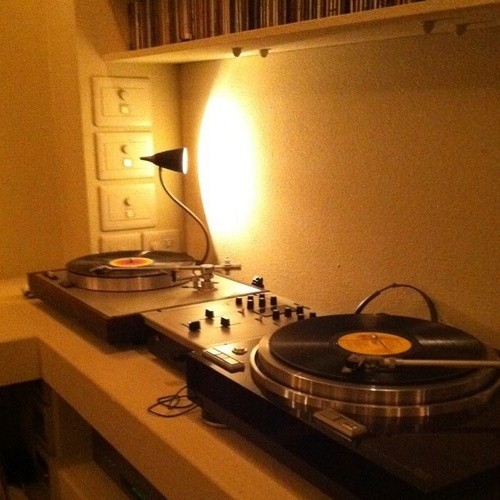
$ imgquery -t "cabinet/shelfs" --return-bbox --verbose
[0,287,343,499]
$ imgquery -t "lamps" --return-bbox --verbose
[140,146,210,267]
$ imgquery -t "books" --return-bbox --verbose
[127,0,417,53]
